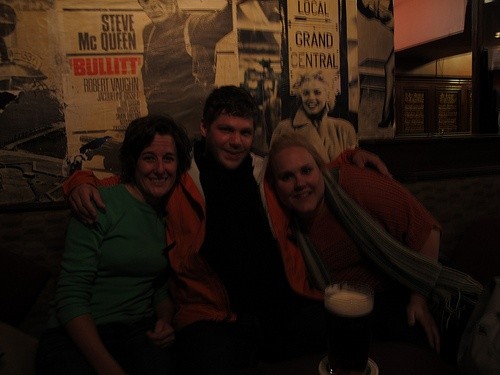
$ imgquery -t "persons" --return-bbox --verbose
[378,46,396,128]
[1,77,37,114]
[54,85,483,375]
[137,0,233,144]
[241,62,268,150]
[270,73,358,163]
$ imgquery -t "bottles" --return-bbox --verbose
[487,32,500,91]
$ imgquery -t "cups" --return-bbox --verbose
[324,281,374,375]
